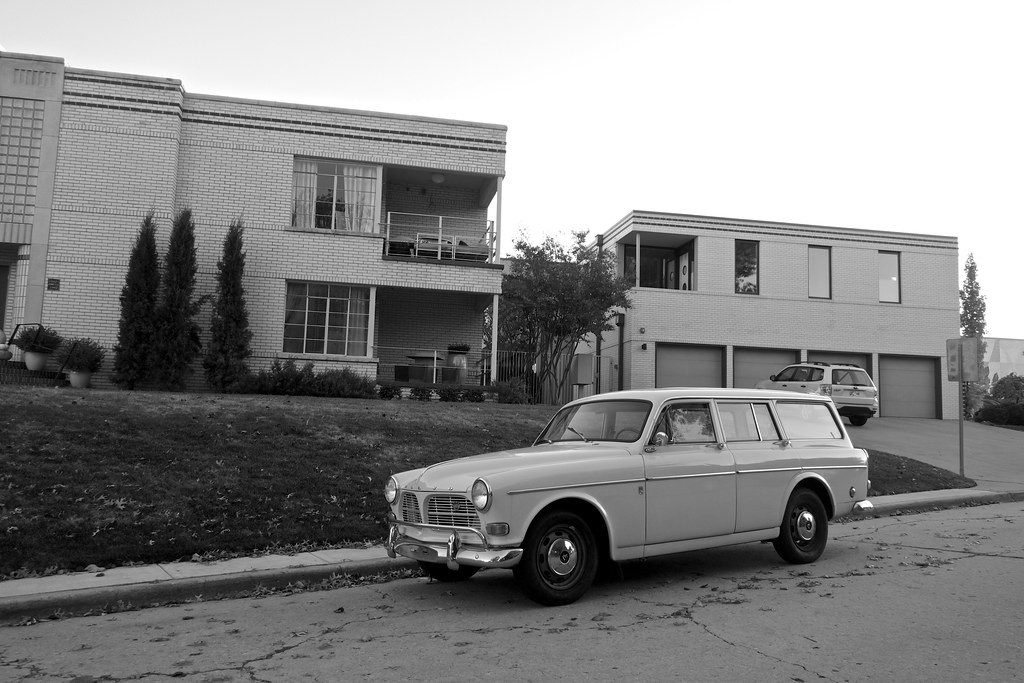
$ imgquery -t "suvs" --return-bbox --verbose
[758,361,880,426]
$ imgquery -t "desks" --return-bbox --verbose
[409,355,446,383]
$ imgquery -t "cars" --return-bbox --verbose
[383,386,871,607]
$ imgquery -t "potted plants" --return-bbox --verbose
[13,325,62,371]
[445,344,472,380]
[56,338,106,390]
